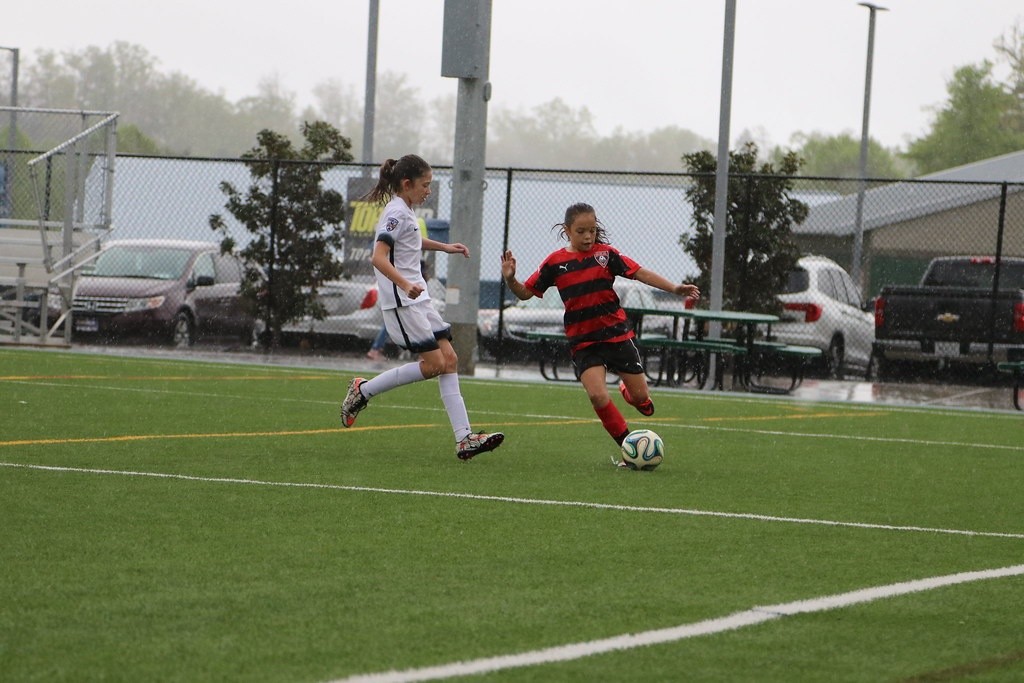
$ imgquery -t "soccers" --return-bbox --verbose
[621,429,665,470]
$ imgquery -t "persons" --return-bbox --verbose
[500,203,701,444]
[340,154,505,460]
[368,260,434,361]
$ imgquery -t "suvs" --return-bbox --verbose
[685,255,875,378]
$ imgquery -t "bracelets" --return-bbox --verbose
[509,279,519,289]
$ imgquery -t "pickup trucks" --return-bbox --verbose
[870,255,1024,380]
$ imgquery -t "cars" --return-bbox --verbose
[45,239,270,348]
[479,280,683,356]
[278,270,448,342]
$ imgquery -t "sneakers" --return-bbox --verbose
[341,378,370,427]
[456,432,505,461]
[619,381,654,416]
[619,460,626,466]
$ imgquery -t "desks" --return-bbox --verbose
[624,305,781,391]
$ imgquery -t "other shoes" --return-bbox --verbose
[368,349,387,360]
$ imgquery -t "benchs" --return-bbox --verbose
[527,329,822,394]
